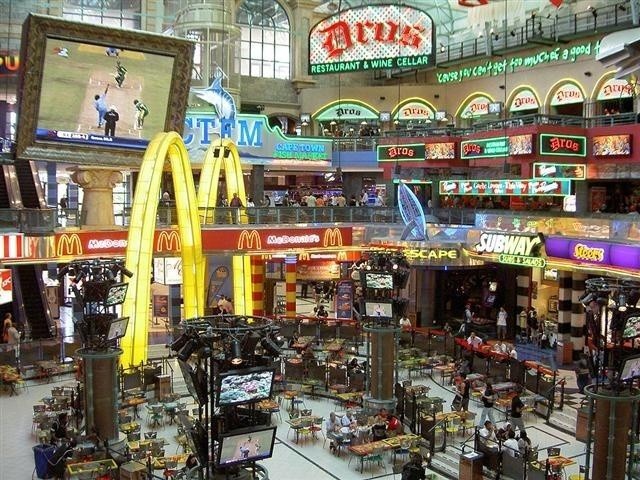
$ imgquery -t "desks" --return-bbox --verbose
[146,402,179,421]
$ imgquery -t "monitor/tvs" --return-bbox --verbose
[57,261,124,307]
[365,302,393,318]
[103,282,129,308]
[616,354,640,385]
[215,369,276,407]
[364,272,396,291]
[32,34,178,154]
[178,413,206,467]
[105,317,130,343]
[176,358,204,408]
[619,312,640,340]
[215,426,277,469]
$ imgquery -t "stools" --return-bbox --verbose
[298,428,310,446]
[446,426,458,447]
[310,427,321,444]
[472,392,482,405]
[461,422,473,440]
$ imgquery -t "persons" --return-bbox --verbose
[375,304,381,318]
[347,358,371,386]
[456,372,469,410]
[496,307,508,339]
[103,105,119,137]
[467,331,482,349]
[103,47,120,58]
[308,306,329,325]
[93,83,110,129]
[326,407,401,449]
[464,305,475,335]
[163,182,386,227]
[479,419,531,457]
[300,280,330,299]
[186,454,198,470]
[478,381,496,425]
[239,435,261,457]
[3,312,21,345]
[401,453,426,480]
[494,340,517,360]
[400,314,411,330]
[509,391,525,435]
[133,99,149,130]
[114,59,128,87]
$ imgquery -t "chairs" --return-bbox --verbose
[166,405,177,425]
[289,411,303,440]
[32,405,46,435]
[402,380,412,386]
[152,406,163,428]
[525,398,537,423]
[133,443,149,460]
[164,461,177,479]
[302,408,312,435]
[151,441,164,457]
[363,447,387,476]
[394,440,410,465]
[321,422,338,452]
